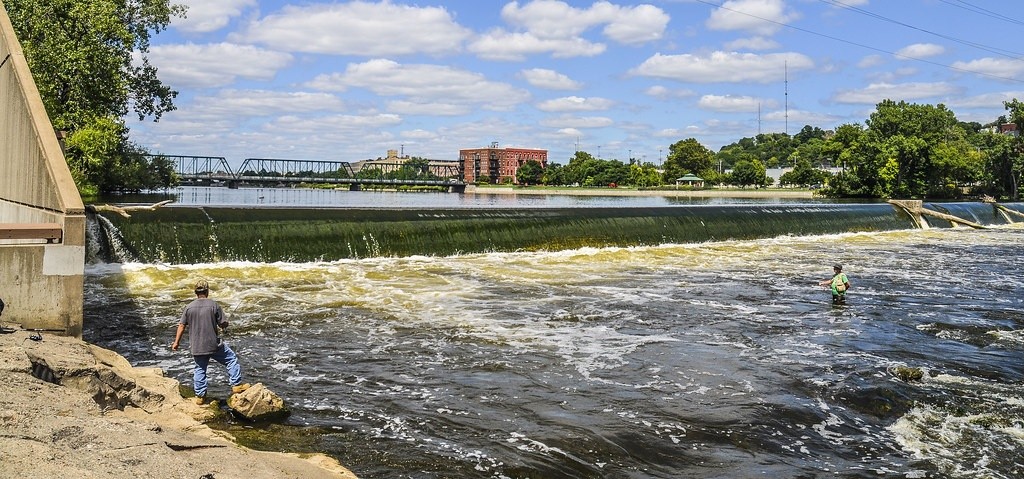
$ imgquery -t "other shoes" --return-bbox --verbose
[196,397,205,404]
[233,382,250,392]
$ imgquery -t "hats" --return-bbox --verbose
[195,280,208,291]
[833,263,842,270]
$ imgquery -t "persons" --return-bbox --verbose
[818,263,850,301]
[170,280,250,405]
